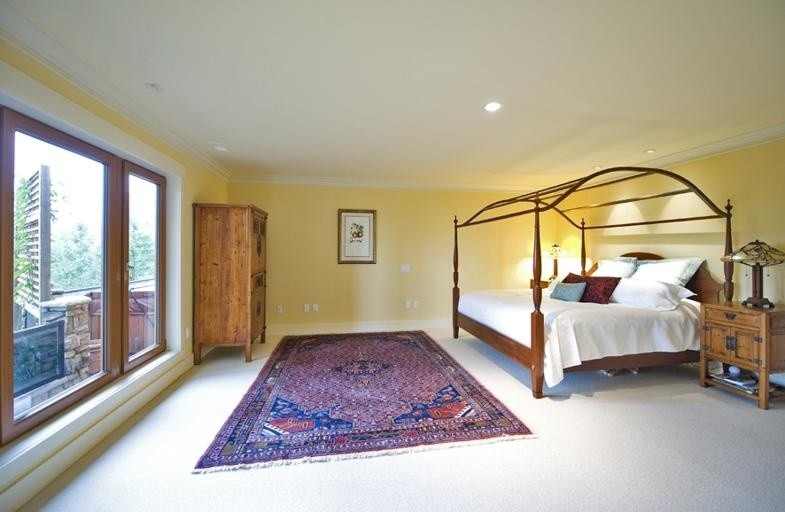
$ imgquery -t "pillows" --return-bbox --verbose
[550,282,586,302]
[609,278,699,312]
[631,257,705,286]
[591,257,638,278]
[561,272,622,304]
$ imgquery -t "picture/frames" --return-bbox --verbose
[338,208,377,264]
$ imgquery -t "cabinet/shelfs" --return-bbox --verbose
[191,201,269,365]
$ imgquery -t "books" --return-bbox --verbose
[712,373,776,395]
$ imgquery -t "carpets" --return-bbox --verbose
[190,331,535,475]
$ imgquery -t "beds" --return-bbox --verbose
[452,166,734,399]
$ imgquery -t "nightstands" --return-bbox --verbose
[700,302,785,410]
[530,279,552,289]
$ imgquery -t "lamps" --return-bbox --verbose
[720,239,785,308]
[548,243,560,280]
